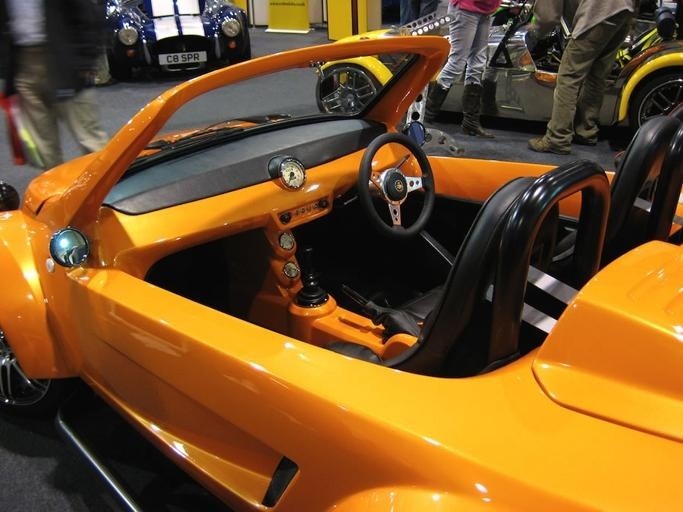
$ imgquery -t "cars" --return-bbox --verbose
[2,34,682,511]
[315,0,682,149]
[105,0,251,81]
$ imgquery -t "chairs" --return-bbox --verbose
[607,6,675,78]
[320,158,611,378]
[554,116,683,270]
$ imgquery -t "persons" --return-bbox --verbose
[0,0,110,172]
[529,0,636,155]
[423,0,502,139]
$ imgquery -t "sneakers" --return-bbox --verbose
[526,133,597,156]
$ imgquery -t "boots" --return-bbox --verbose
[426,81,453,128]
[459,83,494,138]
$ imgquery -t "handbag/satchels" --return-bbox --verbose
[1,92,47,170]
